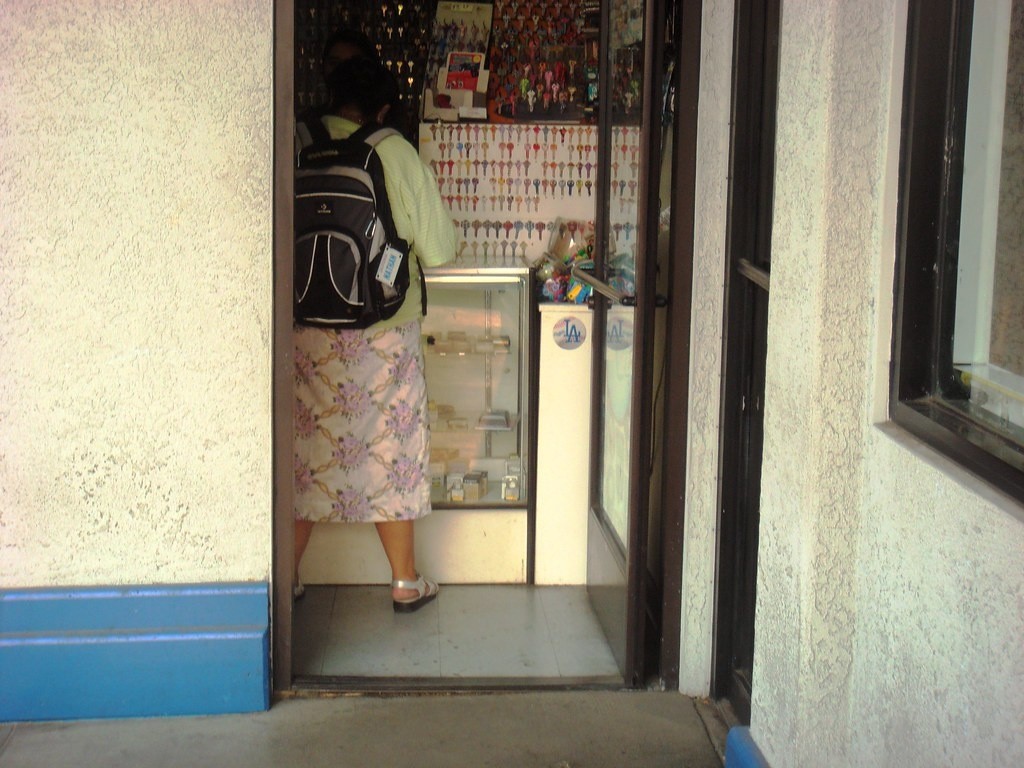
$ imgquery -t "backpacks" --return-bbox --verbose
[293,113,427,329]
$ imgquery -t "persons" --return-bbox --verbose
[293,27,458,614]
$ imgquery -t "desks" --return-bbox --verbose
[532,300,631,588]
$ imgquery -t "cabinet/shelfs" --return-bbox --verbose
[298,265,535,587]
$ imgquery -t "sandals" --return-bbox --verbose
[293,576,305,602]
[392,570,439,613]
